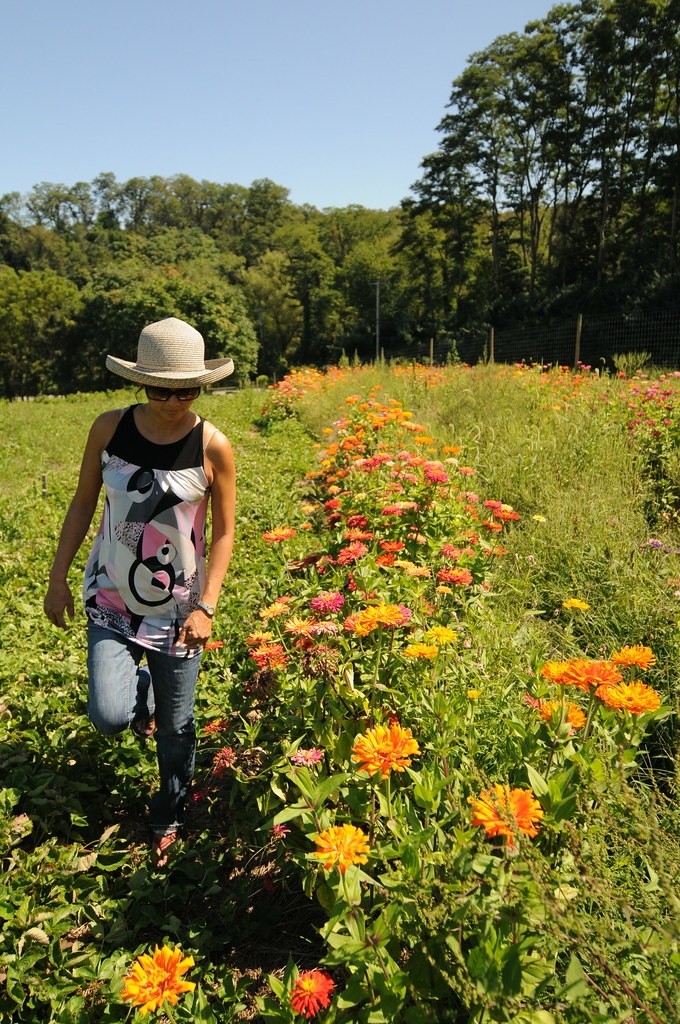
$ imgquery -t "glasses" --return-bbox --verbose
[145,386,201,402]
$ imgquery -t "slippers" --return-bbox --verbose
[151,833,176,869]
[130,716,156,738]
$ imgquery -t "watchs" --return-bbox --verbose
[197,603,216,616]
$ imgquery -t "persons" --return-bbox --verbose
[43,317,236,869]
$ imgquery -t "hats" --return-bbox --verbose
[106,316,235,388]
